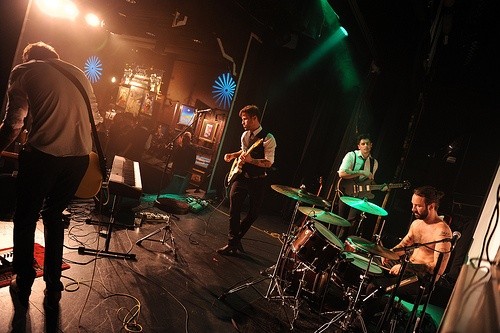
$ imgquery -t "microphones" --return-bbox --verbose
[450,231,461,251]
[196,109,212,113]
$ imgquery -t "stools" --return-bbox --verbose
[135,198,187,259]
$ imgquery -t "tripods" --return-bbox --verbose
[218,198,369,333]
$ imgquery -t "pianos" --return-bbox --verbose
[78,155,143,259]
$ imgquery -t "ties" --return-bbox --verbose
[359,155,369,170]
[248,132,253,139]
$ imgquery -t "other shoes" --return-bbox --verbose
[236,240,243,251]
[217,245,236,254]
[44,288,61,302]
[10,276,32,297]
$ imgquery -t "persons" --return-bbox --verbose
[353,186,452,333]
[0,44,102,302]
[337,135,378,242]
[216,106,276,253]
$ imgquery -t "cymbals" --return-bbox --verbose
[296,207,352,226]
[270,184,332,207]
[345,236,400,263]
[339,195,388,216]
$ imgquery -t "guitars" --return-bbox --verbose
[1,150,105,199]
[337,174,412,200]
[226,137,263,183]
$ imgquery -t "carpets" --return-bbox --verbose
[0,241,71,289]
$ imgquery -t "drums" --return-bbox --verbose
[345,239,371,259]
[288,220,345,273]
[331,253,384,286]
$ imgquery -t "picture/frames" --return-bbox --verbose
[199,119,216,143]
